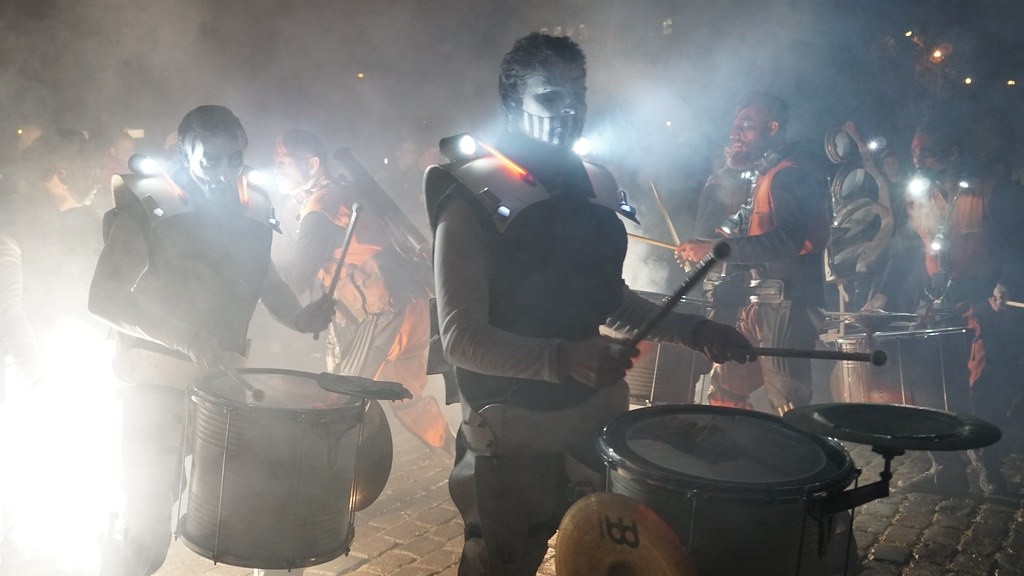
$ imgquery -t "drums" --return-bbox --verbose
[626,287,716,407]
[836,324,971,414]
[593,402,861,576]
[175,367,366,572]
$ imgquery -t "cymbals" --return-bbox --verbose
[553,492,693,576]
[781,402,1002,452]
[347,397,394,513]
[821,306,920,333]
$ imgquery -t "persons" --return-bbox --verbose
[676,92,1024,497]
[422,31,755,576]
[1,106,339,576]
[271,130,461,464]
